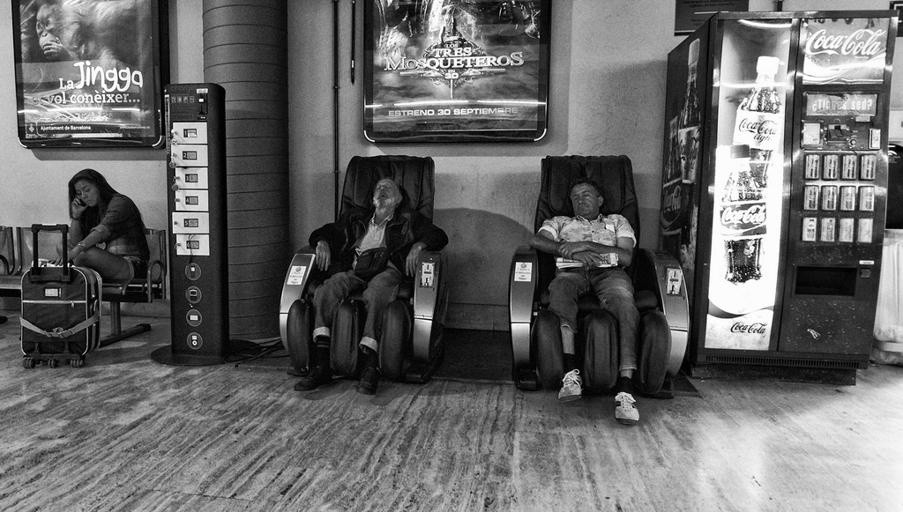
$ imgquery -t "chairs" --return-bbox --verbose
[506,153,683,399]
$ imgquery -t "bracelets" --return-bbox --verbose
[76,241,87,251]
[71,216,80,220]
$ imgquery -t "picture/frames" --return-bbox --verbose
[9,1,169,151]
[359,3,546,145]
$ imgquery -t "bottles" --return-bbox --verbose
[661,118,682,252]
[677,39,700,244]
[720,54,781,242]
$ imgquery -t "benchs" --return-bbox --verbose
[1,225,163,350]
[278,153,449,386]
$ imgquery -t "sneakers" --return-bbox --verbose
[556,366,590,405]
[293,363,337,392]
[614,390,641,428]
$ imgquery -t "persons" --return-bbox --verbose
[528,179,641,427]
[46,169,151,285]
[293,177,449,397]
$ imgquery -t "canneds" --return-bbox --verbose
[803,154,876,245]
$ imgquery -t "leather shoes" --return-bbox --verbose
[351,352,421,395]
